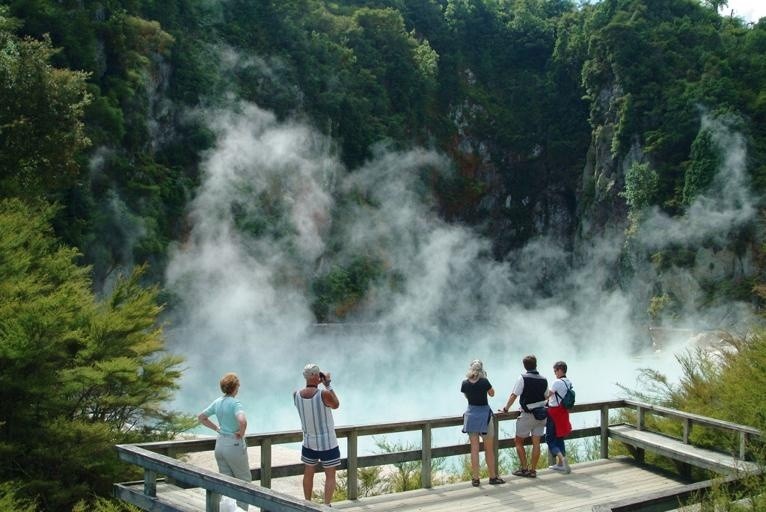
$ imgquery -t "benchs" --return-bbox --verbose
[113,479,206,512]
[609,424,766,480]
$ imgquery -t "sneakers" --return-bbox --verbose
[549,458,570,474]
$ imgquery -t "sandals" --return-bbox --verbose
[529,470,536,477]
[489,477,505,484]
[512,469,529,477]
[471,477,480,486]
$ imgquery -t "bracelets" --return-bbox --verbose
[503,407,508,412]
[325,385,333,391]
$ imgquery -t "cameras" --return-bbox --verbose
[318,371,326,382]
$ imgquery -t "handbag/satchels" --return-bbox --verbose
[532,406,547,420]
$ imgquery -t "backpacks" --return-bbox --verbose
[555,378,575,408]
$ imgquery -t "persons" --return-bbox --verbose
[197,373,254,512]
[291,363,343,507]
[496,354,550,478]
[543,360,577,474]
[459,358,507,486]
